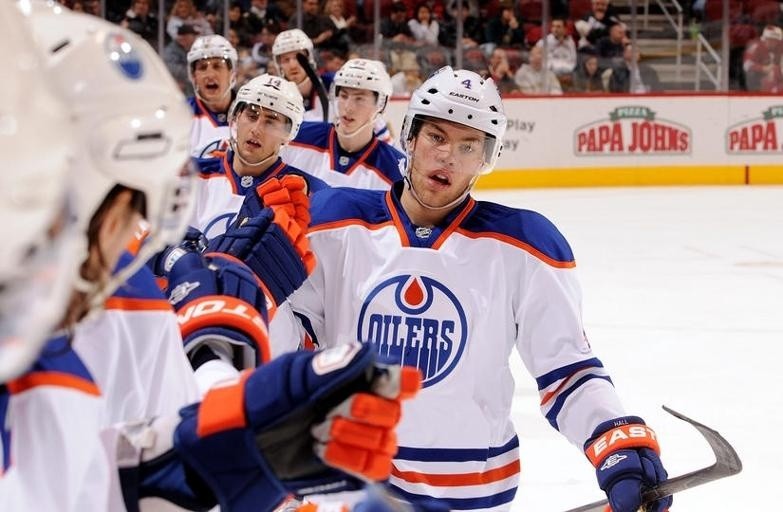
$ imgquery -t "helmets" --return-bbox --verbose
[188,35,237,90]
[333,56,391,112]
[273,29,311,75]
[227,72,302,146]
[400,65,505,175]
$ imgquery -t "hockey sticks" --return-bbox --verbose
[296,52,328,122]
[567,405,743,512]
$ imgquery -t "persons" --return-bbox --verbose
[263,64,680,512]
[1,1,423,512]
[407,1,782,96]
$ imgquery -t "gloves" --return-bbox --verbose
[584,415,673,512]
[172,341,421,512]
[160,175,316,374]
